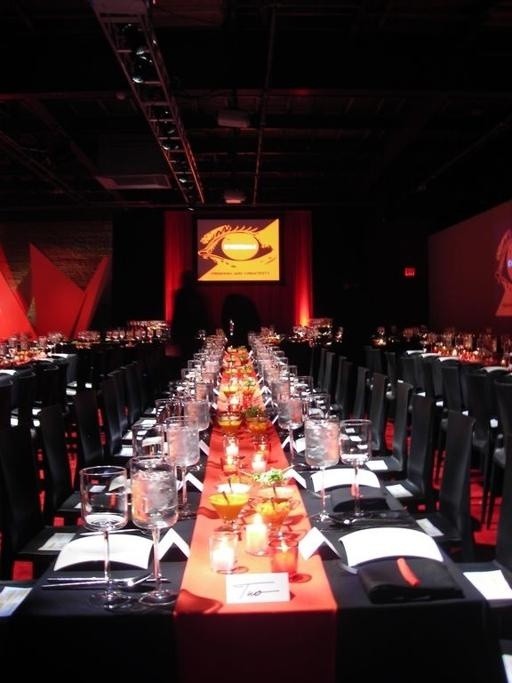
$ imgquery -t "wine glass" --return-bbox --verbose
[0,325,63,371]
[80,328,374,606]
[377,314,494,371]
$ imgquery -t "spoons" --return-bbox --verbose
[40,571,153,592]
[323,509,410,527]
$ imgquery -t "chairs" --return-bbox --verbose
[0,318,511,683]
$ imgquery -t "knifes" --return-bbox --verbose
[48,574,171,586]
